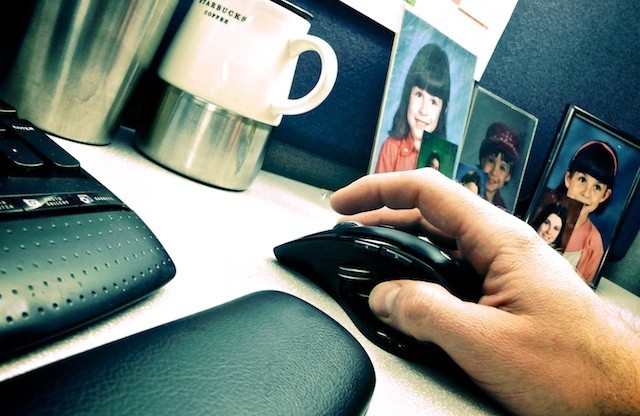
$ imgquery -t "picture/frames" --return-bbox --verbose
[368,0,479,187]
[524,106,640,291]
[457,84,534,216]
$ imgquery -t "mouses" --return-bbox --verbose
[274,221,486,374]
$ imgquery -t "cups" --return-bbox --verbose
[159,0,338,126]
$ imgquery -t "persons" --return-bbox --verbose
[475,121,520,214]
[460,172,480,197]
[423,152,440,170]
[556,138,619,283]
[331,168,639,414]
[373,45,450,171]
[532,203,567,254]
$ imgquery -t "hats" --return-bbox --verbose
[483,122,520,163]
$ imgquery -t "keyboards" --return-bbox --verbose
[0,98,176,361]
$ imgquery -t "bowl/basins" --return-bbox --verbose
[132,84,276,190]
